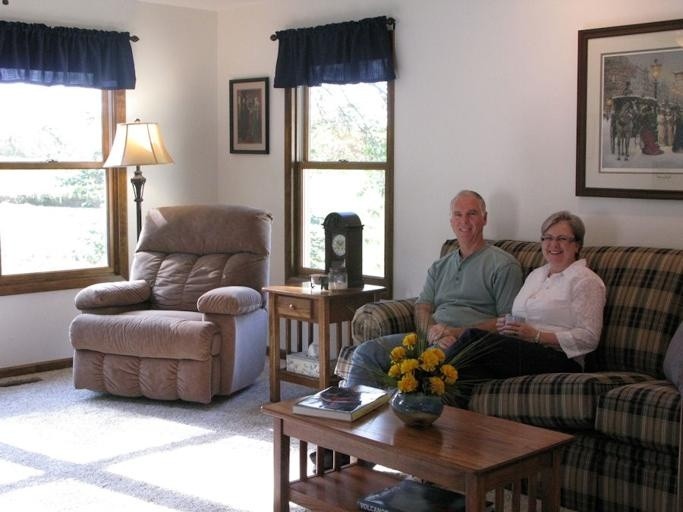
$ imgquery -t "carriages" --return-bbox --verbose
[606,80,659,160]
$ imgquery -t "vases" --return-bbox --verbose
[392,394,443,424]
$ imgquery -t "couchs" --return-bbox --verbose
[350,237,682,511]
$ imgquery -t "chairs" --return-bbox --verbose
[67,205,271,403]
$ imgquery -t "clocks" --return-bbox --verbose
[322,212,362,288]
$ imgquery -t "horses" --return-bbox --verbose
[615,107,635,160]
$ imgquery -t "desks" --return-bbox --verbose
[262,283,387,403]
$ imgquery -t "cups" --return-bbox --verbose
[503,312,526,338]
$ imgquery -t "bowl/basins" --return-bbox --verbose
[310,274,327,290]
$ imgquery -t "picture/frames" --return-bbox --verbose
[227,77,269,154]
[576,19,682,201]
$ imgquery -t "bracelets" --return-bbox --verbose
[533,328,541,343]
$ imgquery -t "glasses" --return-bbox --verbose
[540,235,573,245]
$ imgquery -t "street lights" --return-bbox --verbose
[649,56,662,98]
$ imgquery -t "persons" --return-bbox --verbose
[238,94,260,141]
[310,187,524,476]
[442,210,607,407]
[607,80,682,156]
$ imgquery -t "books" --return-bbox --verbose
[292,384,393,423]
[356,478,493,512]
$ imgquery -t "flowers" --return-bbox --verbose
[377,312,496,408]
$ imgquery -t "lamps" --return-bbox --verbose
[102,117,175,241]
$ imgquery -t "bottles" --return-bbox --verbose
[326,266,348,293]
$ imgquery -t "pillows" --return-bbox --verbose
[661,320,682,387]
